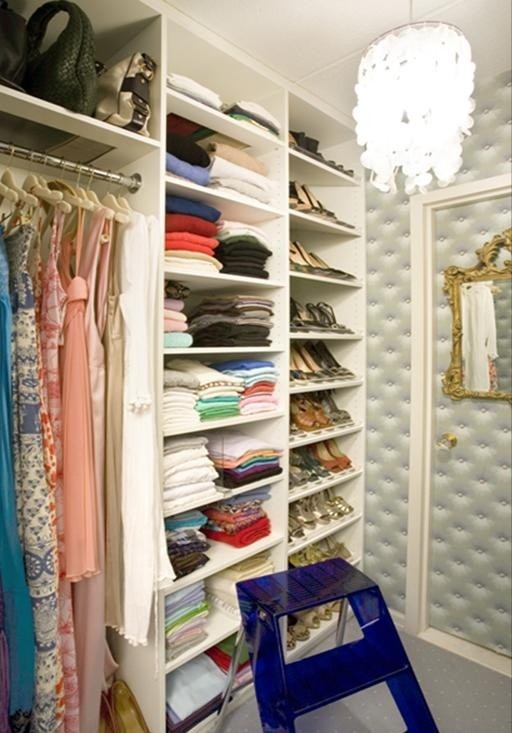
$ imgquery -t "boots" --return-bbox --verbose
[288,130,319,159]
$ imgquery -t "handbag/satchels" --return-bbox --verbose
[92,50,158,141]
[21,0,96,117]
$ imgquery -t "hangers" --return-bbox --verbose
[1,143,161,227]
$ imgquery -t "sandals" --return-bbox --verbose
[288,488,354,544]
[319,153,355,178]
[289,438,352,491]
[288,538,351,569]
[290,392,352,436]
[286,599,342,651]
[288,239,358,280]
[288,180,355,229]
[289,340,355,387]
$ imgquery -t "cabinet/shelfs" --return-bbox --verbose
[0,0,368,732]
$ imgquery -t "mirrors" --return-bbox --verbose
[443,227,512,399]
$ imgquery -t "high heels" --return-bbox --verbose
[290,296,354,335]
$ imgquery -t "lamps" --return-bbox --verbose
[351,0,477,194]
